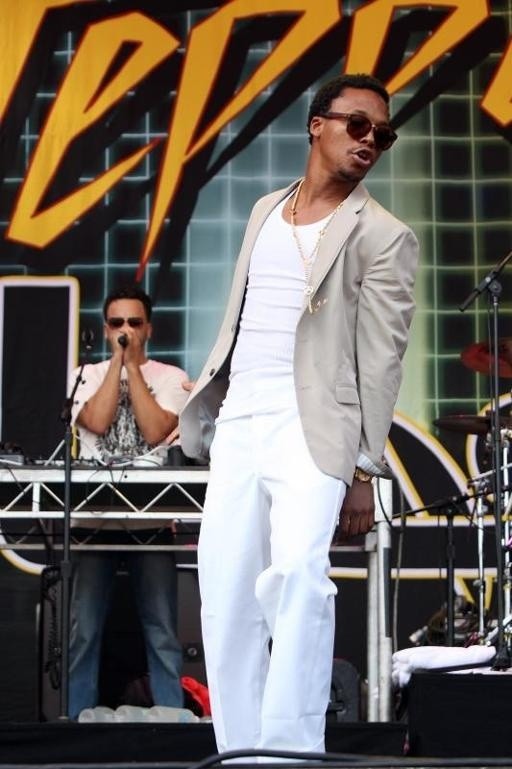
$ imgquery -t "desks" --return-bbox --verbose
[0,463,393,725]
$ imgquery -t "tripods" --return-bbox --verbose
[423,254,512,677]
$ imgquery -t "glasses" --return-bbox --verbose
[315,109,399,151]
[106,316,146,329]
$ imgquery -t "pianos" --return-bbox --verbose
[0,466,389,554]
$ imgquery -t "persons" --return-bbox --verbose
[60,283,195,722]
[177,68,424,769]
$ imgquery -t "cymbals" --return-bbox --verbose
[461,334,510,377]
[432,414,511,437]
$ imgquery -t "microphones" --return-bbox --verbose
[117,336,128,347]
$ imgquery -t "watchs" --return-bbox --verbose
[352,466,376,485]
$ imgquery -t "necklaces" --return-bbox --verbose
[288,178,350,317]
